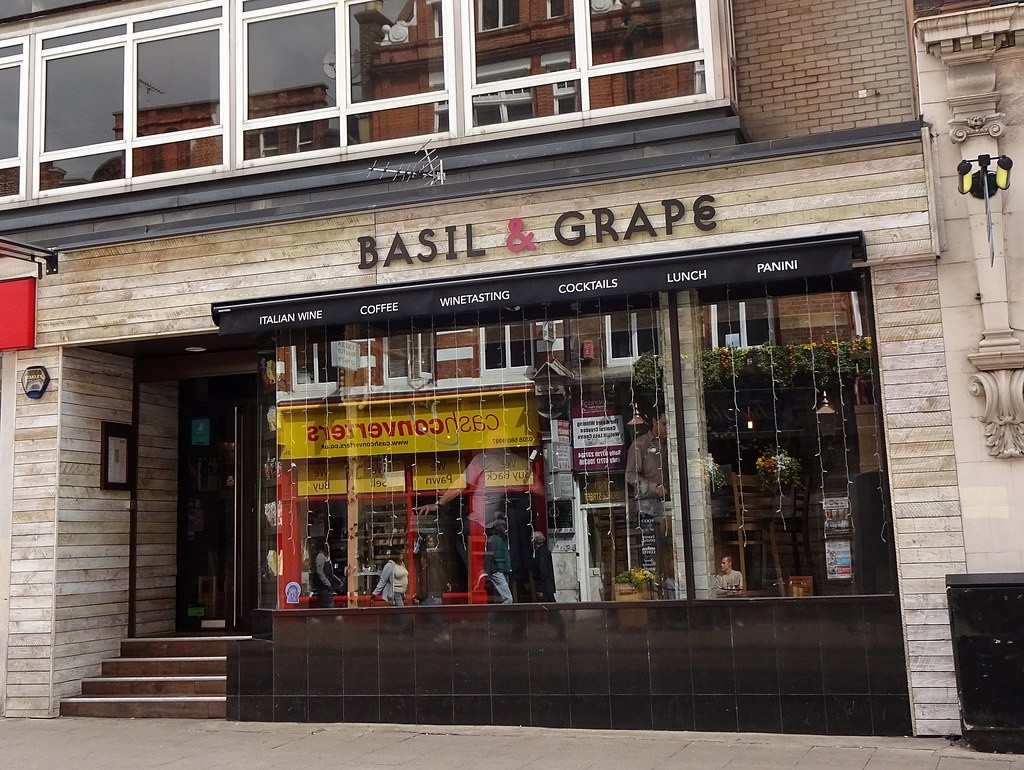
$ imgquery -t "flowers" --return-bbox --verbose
[755,446,804,496]
[633,337,874,390]
[613,566,657,585]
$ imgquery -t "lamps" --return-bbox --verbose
[627,401,649,426]
[991,155,1013,191]
[816,385,838,437]
[956,159,979,195]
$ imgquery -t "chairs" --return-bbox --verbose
[589,500,668,599]
[759,475,820,594]
[789,576,813,597]
[719,473,789,599]
[362,498,430,597]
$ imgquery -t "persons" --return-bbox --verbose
[370,543,409,606]
[414,533,451,605]
[482,528,511,604]
[310,541,343,607]
[666,575,675,598]
[715,555,743,594]
[624,412,668,601]
[527,531,557,601]
[414,446,546,604]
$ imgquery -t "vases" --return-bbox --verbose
[614,581,655,601]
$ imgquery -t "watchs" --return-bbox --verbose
[434,500,443,508]
